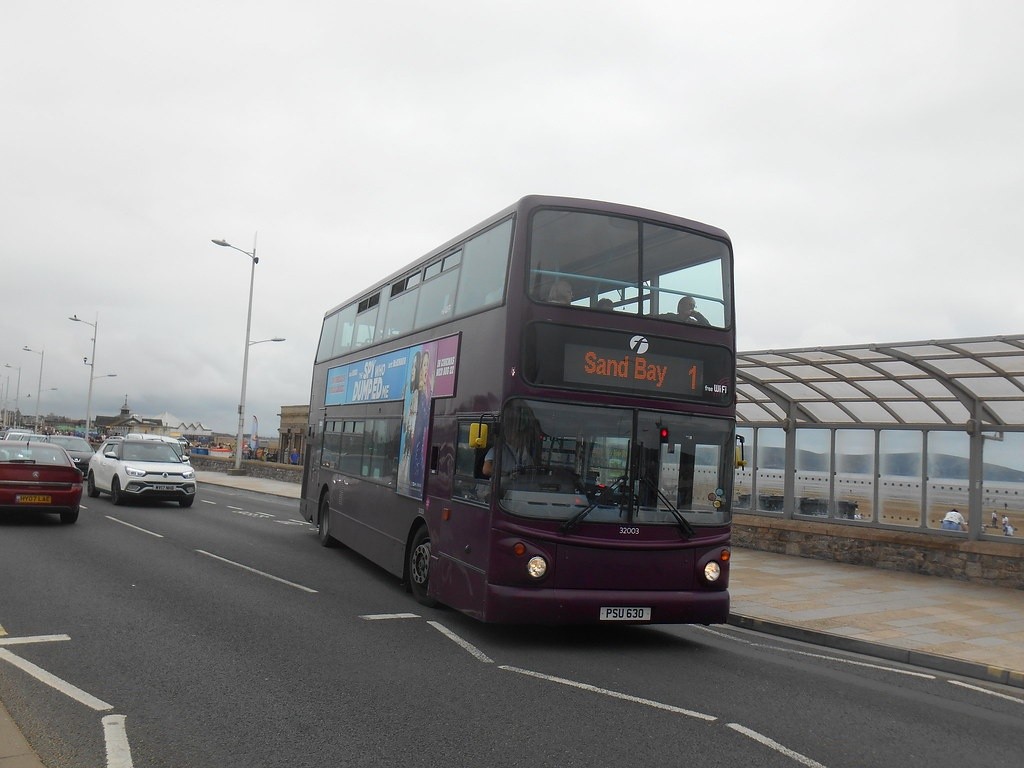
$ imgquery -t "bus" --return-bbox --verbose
[298,192,747,627]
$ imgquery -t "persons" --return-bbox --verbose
[289,448,299,465]
[548,271,710,325]
[942,501,1017,536]
[190,441,268,462]
[483,424,535,482]
[0,419,120,443]
[397,350,431,495]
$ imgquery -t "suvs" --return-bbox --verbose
[86,435,197,507]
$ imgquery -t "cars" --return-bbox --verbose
[0,426,48,444]
[40,435,96,478]
[0,440,83,524]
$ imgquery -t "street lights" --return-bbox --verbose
[22,345,58,433]
[4,363,32,430]
[68,311,118,440]
[210,228,287,477]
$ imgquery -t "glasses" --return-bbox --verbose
[684,303,697,308]
